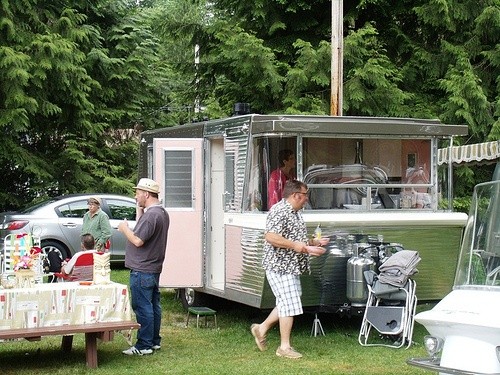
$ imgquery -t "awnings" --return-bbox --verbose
[437,140,500,166]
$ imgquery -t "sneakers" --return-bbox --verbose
[152,344,161,351]
[122,346,153,356]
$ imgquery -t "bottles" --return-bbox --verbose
[315,222,322,240]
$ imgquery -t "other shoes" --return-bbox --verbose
[276,346,303,358]
[251,323,267,352]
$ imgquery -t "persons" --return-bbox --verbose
[80,194,113,251]
[249,179,324,359]
[117,177,170,355]
[57,233,103,284]
[266,148,298,210]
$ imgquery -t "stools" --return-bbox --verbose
[186,307,217,330]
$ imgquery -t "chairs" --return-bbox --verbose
[359,274,419,348]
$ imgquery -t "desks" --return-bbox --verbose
[1,321,141,370]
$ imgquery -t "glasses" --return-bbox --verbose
[292,191,307,196]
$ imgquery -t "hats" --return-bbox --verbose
[132,178,160,193]
[89,196,101,204]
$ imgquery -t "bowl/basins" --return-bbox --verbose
[320,238,331,245]
[307,246,326,257]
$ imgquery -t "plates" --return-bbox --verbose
[343,204,382,209]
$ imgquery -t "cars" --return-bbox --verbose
[0,193,138,276]
